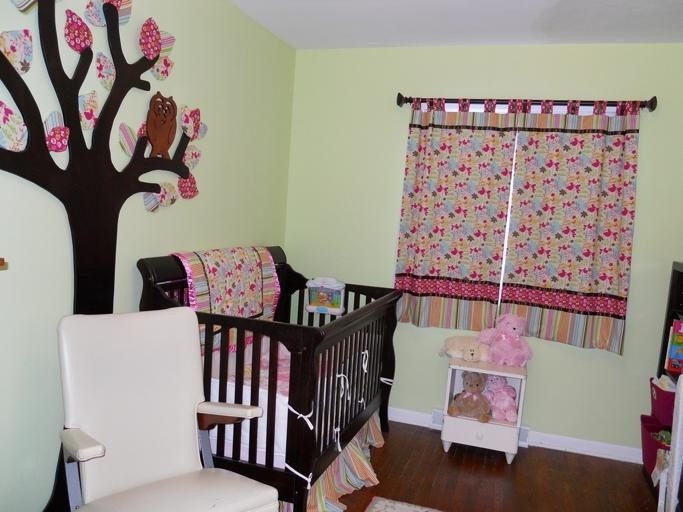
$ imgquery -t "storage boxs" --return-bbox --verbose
[640,413,670,487]
[649,373,681,424]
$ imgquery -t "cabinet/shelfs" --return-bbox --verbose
[441,363,527,467]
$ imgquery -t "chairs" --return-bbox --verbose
[54,303,278,512]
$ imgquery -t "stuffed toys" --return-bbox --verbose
[304,276,345,315]
[481,375,518,424]
[437,336,489,363]
[663,319,683,377]
[447,371,490,423]
[480,314,533,368]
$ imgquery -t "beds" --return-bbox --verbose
[134,244,404,512]
